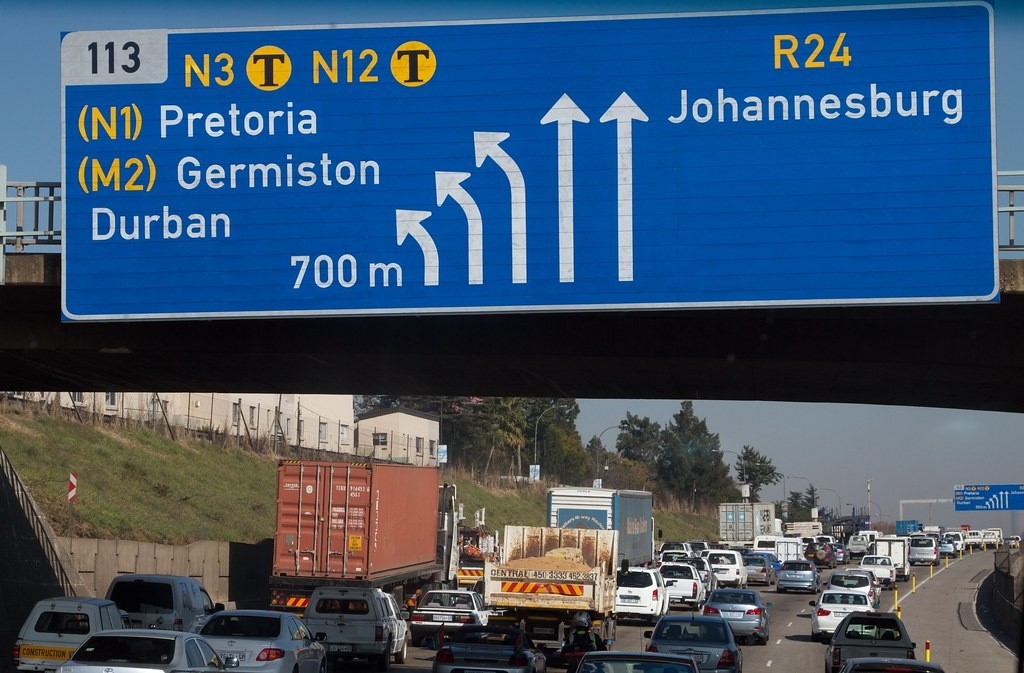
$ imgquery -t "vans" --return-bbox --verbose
[14,574,226,673]
[754,535,780,558]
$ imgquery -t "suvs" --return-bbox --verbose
[805,543,837,569]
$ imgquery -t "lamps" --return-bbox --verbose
[195,401,200,407]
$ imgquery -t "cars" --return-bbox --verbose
[615,535,870,645]
[909,527,1021,566]
[810,554,944,673]
[409,577,548,673]
[572,613,742,673]
[56,611,330,673]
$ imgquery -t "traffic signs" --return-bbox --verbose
[60,2,1000,320]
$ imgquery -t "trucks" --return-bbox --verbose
[268,459,501,616]
[484,525,630,656]
[895,520,946,537]
[718,502,788,550]
[774,539,807,563]
[547,487,662,569]
[875,538,912,582]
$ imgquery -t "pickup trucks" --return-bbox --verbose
[303,587,408,667]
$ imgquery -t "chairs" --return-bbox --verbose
[834,581,844,586]
[847,631,860,639]
[716,596,727,602]
[663,667,679,673]
[852,596,862,603]
[858,578,867,586]
[741,595,752,603]
[59,614,78,629]
[881,631,895,641]
[432,599,443,606]
[696,561,704,570]
[665,624,682,639]
[881,560,887,564]
[702,626,720,640]
[670,546,675,549]
[668,571,674,576]
[826,594,837,603]
[503,634,518,644]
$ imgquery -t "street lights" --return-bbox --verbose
[534,404,567,481]
[757,463,785,500]
[712,450,746,500]
[596,423,627,484]
[787,475,881,529]
